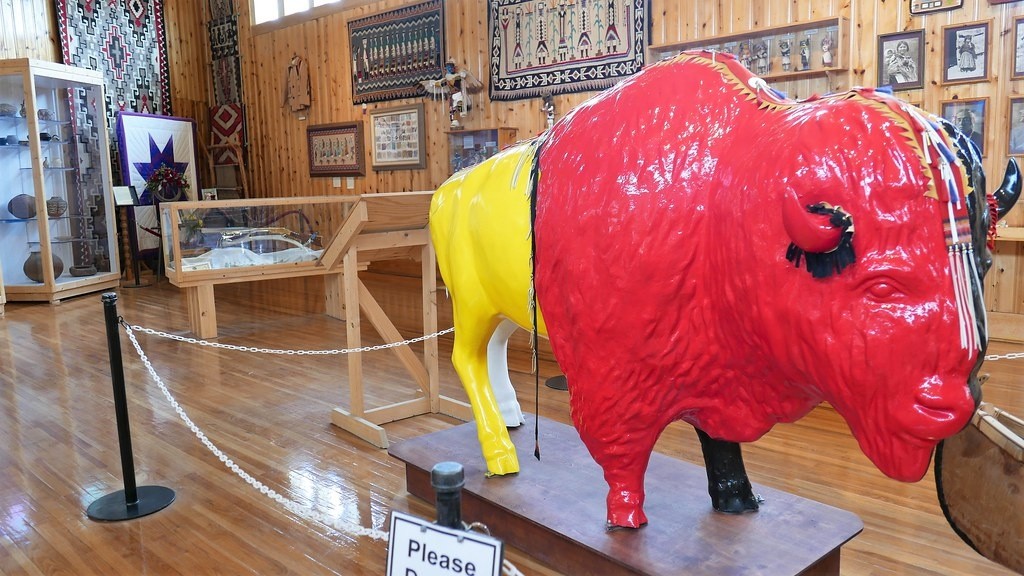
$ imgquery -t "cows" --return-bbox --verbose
[425,50,1024,529]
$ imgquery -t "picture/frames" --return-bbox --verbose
[1006,94,1024,157]
[307,119,365,177]
[1010,15,1023,80]
[369,104,427,170]
[940,18,996,85]
[939,97,990,158]
[876,29,926,91]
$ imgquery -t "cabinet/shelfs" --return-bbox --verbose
[1,56,120,306]
[445,126,519,177]
[646,15,849,82]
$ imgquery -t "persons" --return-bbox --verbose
[953,108,982,148]
[959,35,977,72]
[884,41,918,84]
